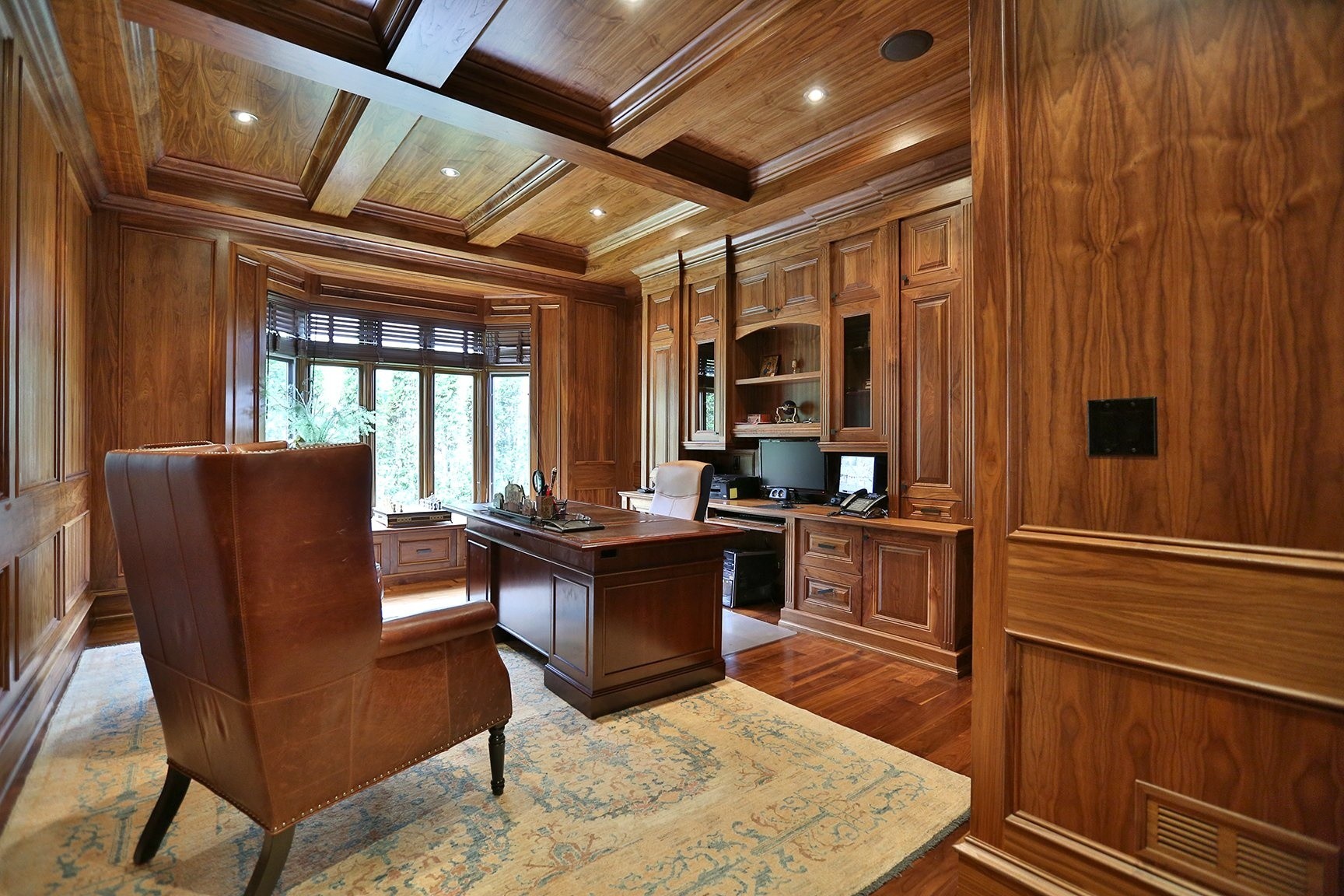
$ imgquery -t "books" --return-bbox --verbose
[543,519,605,533]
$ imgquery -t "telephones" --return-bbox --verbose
[840,488,889,519]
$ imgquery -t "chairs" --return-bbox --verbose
[648,460,714,523]
[104,439,513,896]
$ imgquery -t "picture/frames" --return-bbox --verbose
[760,354,781,377]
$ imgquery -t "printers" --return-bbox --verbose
[710,475,747,500]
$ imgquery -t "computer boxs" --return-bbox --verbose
[722,548,776,608]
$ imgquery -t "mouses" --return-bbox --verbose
[781,504,793,508]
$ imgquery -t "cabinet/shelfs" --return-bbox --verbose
[617,175,973,680]
[371,511,468,586]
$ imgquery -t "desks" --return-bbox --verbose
[441,499,745,720]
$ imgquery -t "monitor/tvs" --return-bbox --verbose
[759,438,828,503]
[836,454,877,496]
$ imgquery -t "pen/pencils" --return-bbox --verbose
[561,499,568,509]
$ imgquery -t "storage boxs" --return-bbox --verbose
[748,414,772,424]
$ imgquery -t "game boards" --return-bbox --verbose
[372,504,452,528]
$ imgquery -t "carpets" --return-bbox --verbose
[721,606,798,657]
[0,585,972,896]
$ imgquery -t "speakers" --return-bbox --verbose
[769,487,791,500]
